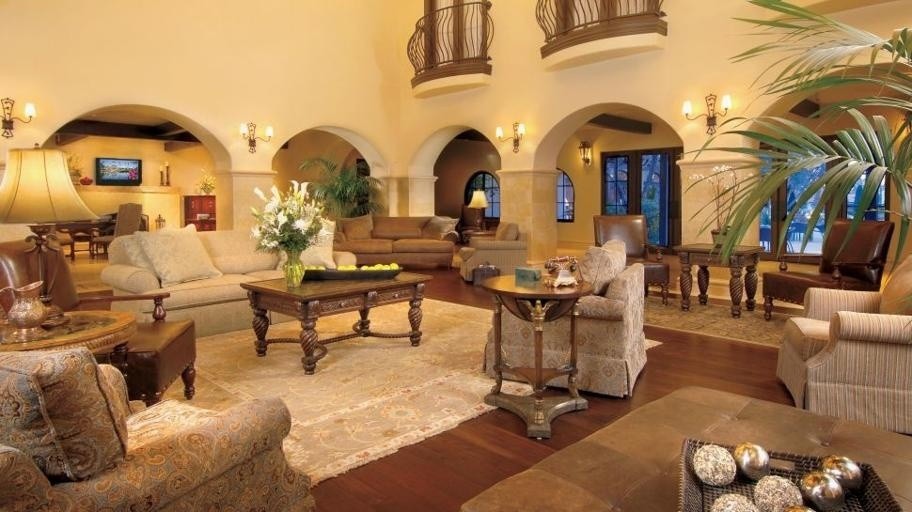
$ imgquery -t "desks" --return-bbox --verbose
[458,386,911,512]
[674,242,767,318]
[482,274,590,443]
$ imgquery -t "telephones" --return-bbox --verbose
[544,255,579,287]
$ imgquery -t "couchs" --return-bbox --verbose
[113,221,360,337]
[330,209,459,273]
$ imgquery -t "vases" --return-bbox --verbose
[281,245,306,288]
[710,227,731,250]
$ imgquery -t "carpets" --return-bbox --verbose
[129,293,549,489]
[644,293,802,350]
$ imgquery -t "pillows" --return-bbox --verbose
[276,222,339,275]
[340,211,458,242]
[137,224,222,291]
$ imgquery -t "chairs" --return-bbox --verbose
[596,214,671,308]
[481,238,648,400]
[459,220,530,282]
[762,215,912,436]
[51,200,143,262]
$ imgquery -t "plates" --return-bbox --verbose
[303,269,402,279]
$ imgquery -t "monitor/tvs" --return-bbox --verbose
[95,157,143,186]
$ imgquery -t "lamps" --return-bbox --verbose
[239,117,274,154]
[468,188,490,233]
[681,92,733,135]
[494,120,526,153]
[0,142,101,332]
[577,140,594,167]
[0,97,36,139]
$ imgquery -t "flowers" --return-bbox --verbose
[688,164,756,232]
[244,178,327,284]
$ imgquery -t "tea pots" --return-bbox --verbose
[0,281,53,341]
